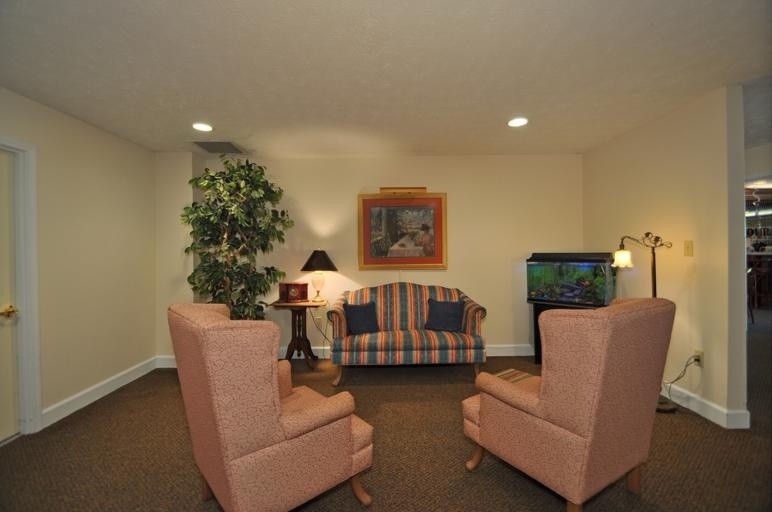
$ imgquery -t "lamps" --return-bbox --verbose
[611,232,678,413]
[300,250,338,303]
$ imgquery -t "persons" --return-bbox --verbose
[415,224,433,256]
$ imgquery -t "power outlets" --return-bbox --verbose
[692,349,704,367]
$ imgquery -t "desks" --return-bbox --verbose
[269,300,326,369]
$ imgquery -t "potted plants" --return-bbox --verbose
[180,149,292,320]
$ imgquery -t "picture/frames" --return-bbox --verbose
[357,192,447,271]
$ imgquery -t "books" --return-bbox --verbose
[279,284,308,302]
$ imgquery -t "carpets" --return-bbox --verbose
[492,368,532,384]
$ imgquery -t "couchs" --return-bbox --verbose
[327,281,486,387]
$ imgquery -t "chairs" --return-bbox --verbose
[747,268,755,325]
[166,302,374,512]
[462,297,675,512]
[748,253,772,309]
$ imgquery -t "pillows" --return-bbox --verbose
[342,301,380,335]
[425,298,464,333]
